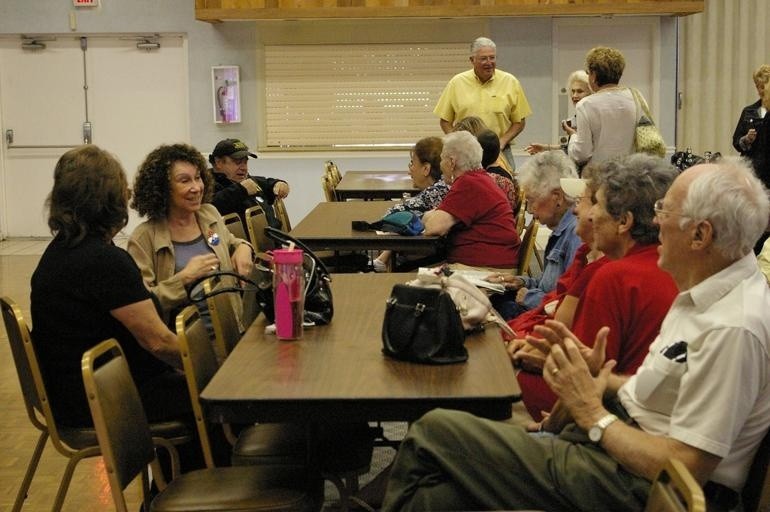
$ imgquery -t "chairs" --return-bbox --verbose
[222,212,247,241]
[175,304,375,512]
[516,186,527,236]
[203,270,248,360]
[645,457,706,512]
[320,160,347,202]
[81,337,313,511]
[518,218,540,283]
[0,296,192,512]
[273,197,291,233]
[244,205,274,255]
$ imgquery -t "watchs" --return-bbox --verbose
[588,414,617,445]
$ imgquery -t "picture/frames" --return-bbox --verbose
[211,65,241,124]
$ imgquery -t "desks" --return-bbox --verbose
[199,272,523,512]
[287,201,442,272]
[335,170,454,202]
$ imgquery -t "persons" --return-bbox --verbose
[29,145,234,510]
[473,130,515,179]
[732,65,770,258]
[431,36,532,171]
[506,155,682,423]
[128,144,254,365]
[366,138,454,270]
[523,70,591,157]
[484,150,584,320]
[452,117,515,174]
[562,46,650,177]
[203,137,289,261]
[422,129,521,278]
[345,157,767,512]
[499,148,630,345]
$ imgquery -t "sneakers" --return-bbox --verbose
[359,260,387,273]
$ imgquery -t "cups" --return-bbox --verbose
[274,249,304,340]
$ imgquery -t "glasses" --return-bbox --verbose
[654,199,693,219]
[126,187,132,202]
[525,195,543,203]
[574,196,591,206]
[477,56,496,62]
[409,160,417,169]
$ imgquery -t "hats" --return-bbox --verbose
[212,139,257,160]
[559,179,597,202]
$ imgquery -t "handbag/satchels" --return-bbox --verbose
[629,88,666,159]
[381,284,469,365]
[187,225,333,326]
[671,147,722,173]
[405,270,517,339]
[352,210,426,235]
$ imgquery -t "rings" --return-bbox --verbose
[211,266,215,270]
[548,369,558,375]
